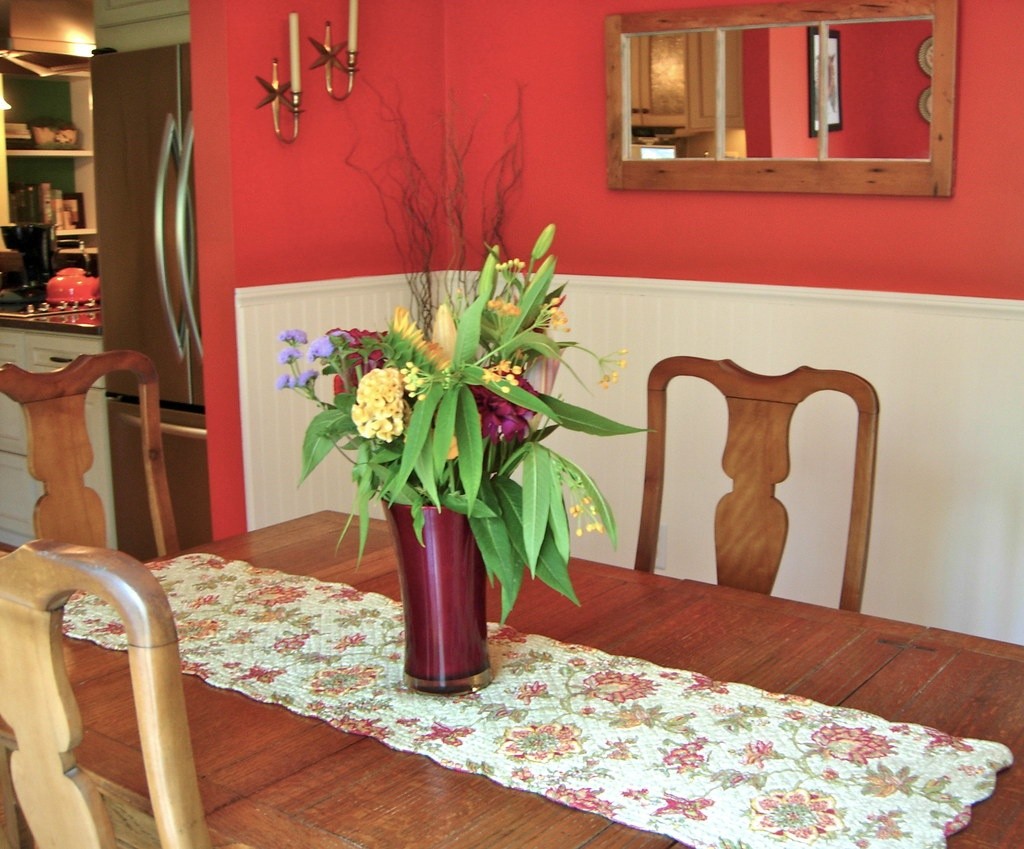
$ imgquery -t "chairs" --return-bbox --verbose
[0,535,210,848]
[0,349,183,558]
[632,355,882,614]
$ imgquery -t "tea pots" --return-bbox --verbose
[44,245,100,304]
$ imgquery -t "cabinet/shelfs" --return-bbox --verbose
[0,0,95,58]
[1,50,97,257]
[91,0,192,54]
[0,328,119,554]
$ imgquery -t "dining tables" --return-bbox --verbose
[6,508,1024,847]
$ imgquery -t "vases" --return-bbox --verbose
[380,495,495,699]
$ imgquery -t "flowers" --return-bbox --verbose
[271,222,662,631]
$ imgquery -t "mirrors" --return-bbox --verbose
[602,2,960,200]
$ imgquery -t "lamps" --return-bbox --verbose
[307,1,361,102]
[254,10,307,144]
